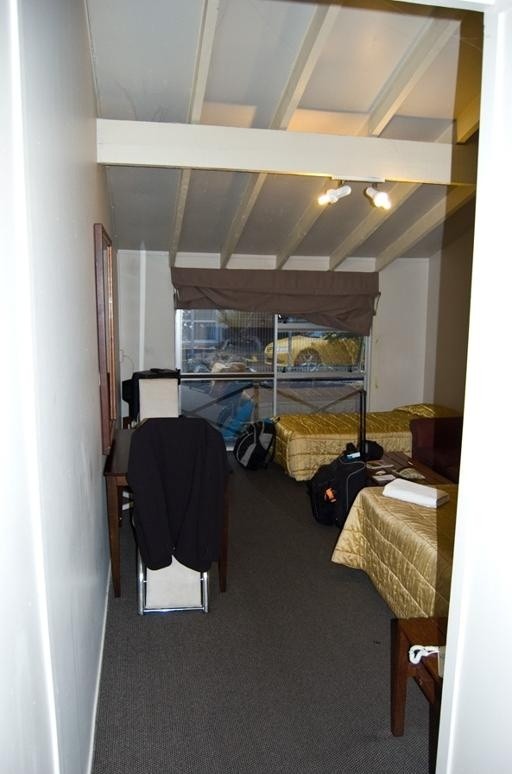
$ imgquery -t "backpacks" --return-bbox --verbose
[307,442,367,531]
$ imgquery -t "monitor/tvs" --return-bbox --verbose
[122,368,180,421]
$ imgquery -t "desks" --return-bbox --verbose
[102,427,242,598]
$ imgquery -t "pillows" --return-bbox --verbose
[396,402,460,421]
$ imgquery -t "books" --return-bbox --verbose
[367,460,393,468]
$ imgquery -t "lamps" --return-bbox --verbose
[317,176,392,210]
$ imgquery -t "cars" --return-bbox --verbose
[264,329,365,373]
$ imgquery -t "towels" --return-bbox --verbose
[381,477,450,508]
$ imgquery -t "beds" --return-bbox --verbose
[267,404,462,480]
[352,484,458,619]
